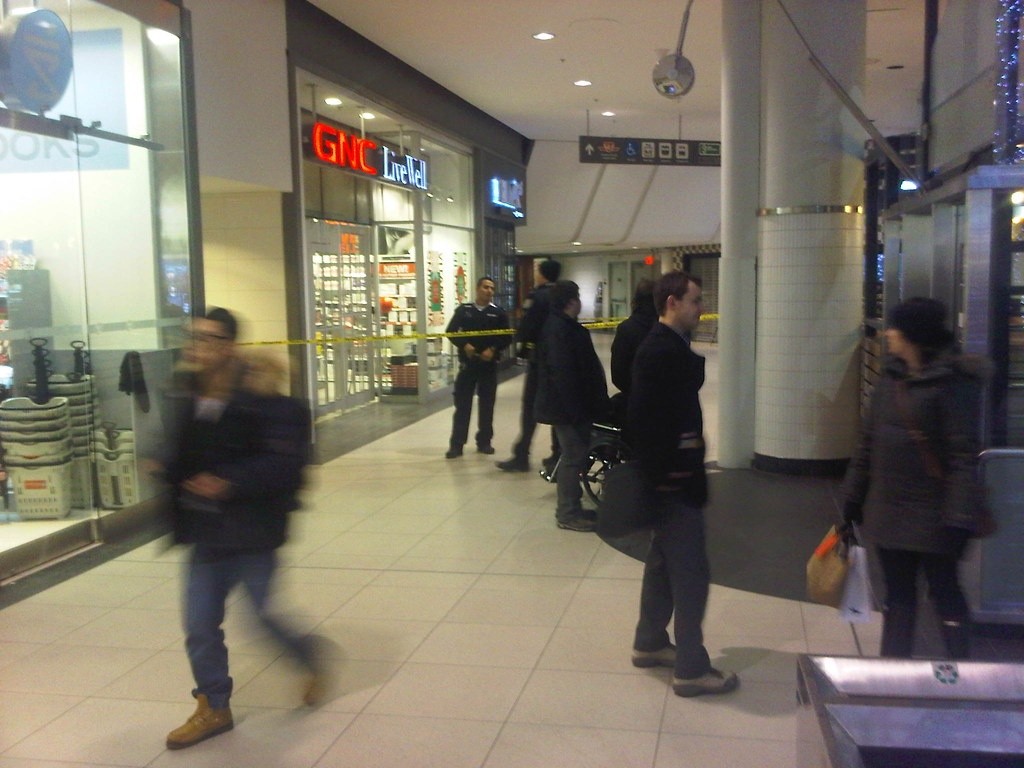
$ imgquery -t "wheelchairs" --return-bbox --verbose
[540,395,624,506]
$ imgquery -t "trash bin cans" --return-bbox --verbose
[794,653,1023,767]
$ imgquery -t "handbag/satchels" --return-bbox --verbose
[840,520,872,624]
[806,524,850,603]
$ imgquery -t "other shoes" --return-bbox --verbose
[557,516,599,531]
[165,694,233,749]
[543,458,557,473]
[497,455,529,472]
[554,509,597,520]
[477,443,494,453]
[305,644,351,707]
[446,444,462,458]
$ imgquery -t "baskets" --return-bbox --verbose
[0,337,140,520]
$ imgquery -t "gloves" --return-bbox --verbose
[942,525,972,547]
[842,498,865,530]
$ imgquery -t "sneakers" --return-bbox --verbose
[631,644,677,669]
[674,667,737,698]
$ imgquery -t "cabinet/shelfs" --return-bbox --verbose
[368,294,419,340]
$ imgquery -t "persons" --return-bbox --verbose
[534,279,610,533]
[496,260,563,472]
[151,308,326,748]
[841,295,992,661]
[446,278,512,459]
[611,268,737,698]
